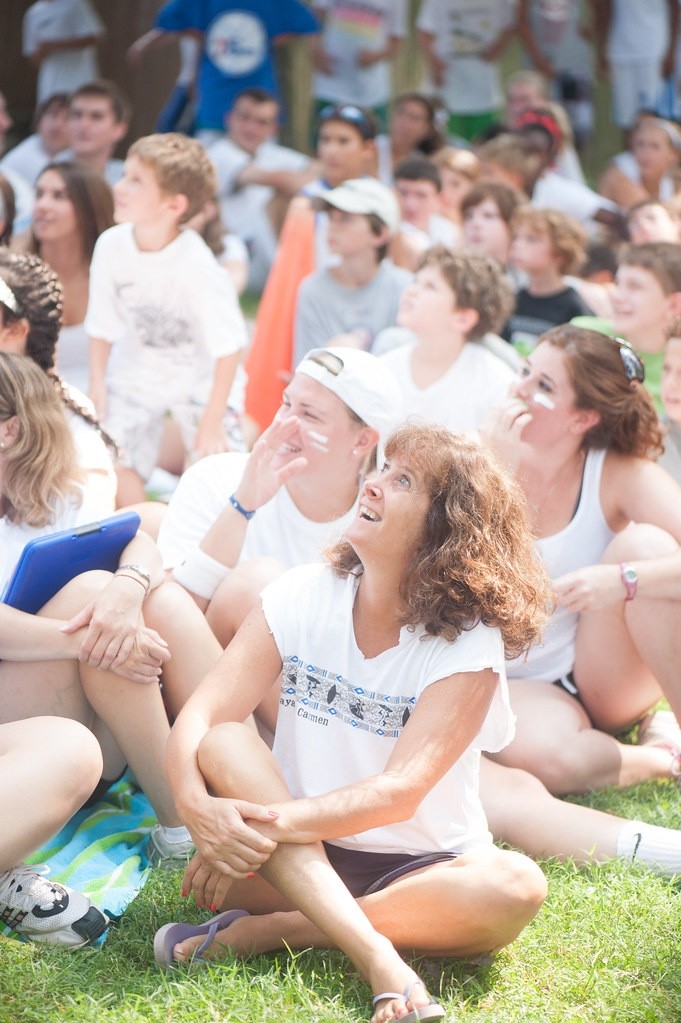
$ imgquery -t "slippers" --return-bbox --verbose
[372,980,445,1023]
[154,910,251,973]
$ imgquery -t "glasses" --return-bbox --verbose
[569,313,645,384]
[320,104,369,135]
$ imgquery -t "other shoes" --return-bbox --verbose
[638,709,681,777]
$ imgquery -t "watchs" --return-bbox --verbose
[620,563,638,601]
[229,495,255,520]
[115,563,151,598]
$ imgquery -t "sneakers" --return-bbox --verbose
[146,824,197,870]
[0,862,109,953]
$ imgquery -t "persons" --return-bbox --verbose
[0,0,681,1023]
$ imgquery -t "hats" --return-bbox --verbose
[298,347,393,434]
[311,176,400,237]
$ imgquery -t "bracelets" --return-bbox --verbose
[115,574,147,593]
